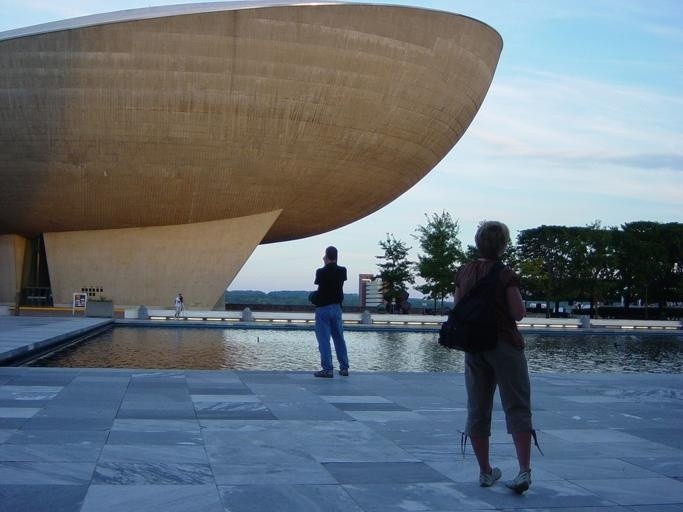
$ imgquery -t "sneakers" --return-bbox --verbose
[479,467,501,487]
[314,369,333,378]
[505,471,531,492]
[339,370,349,376]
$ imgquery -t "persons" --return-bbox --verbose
[314,246,349,378]
[453,220,533,494]
[174,292,184,317]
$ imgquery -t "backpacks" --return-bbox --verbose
[438,261,505,352]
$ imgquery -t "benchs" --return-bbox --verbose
[8,306,124,320]
